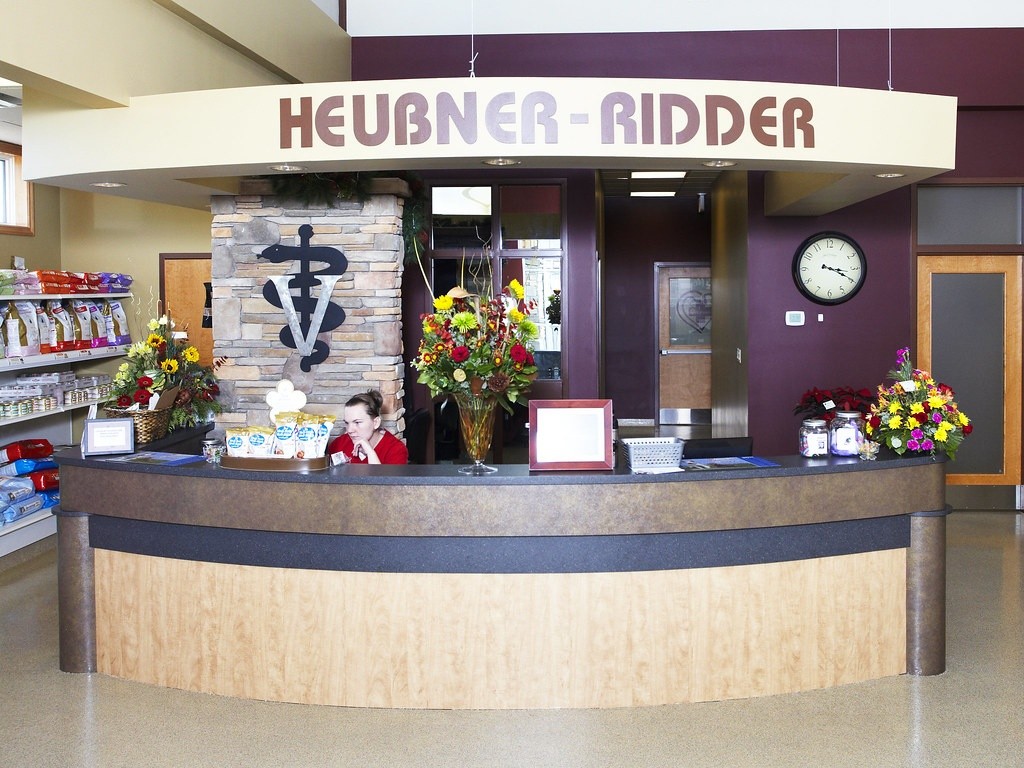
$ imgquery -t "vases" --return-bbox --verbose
[453,390,498,474]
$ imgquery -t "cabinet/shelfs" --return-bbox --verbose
[0,292,134,556]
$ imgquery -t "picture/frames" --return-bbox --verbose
[84,417,135,457]
[527,399,616,473]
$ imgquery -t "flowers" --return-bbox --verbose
[113,318,228,420]
[410,278,539,410]
[865,347,973,461]
[792,385,873,425]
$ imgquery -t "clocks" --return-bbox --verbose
[793,232,867,306]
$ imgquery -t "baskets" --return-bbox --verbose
[103,404,170,443]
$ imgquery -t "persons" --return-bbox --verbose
[325,388,408,465]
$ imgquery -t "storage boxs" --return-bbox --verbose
[103,403,172,444]
[620,437,687,470]
